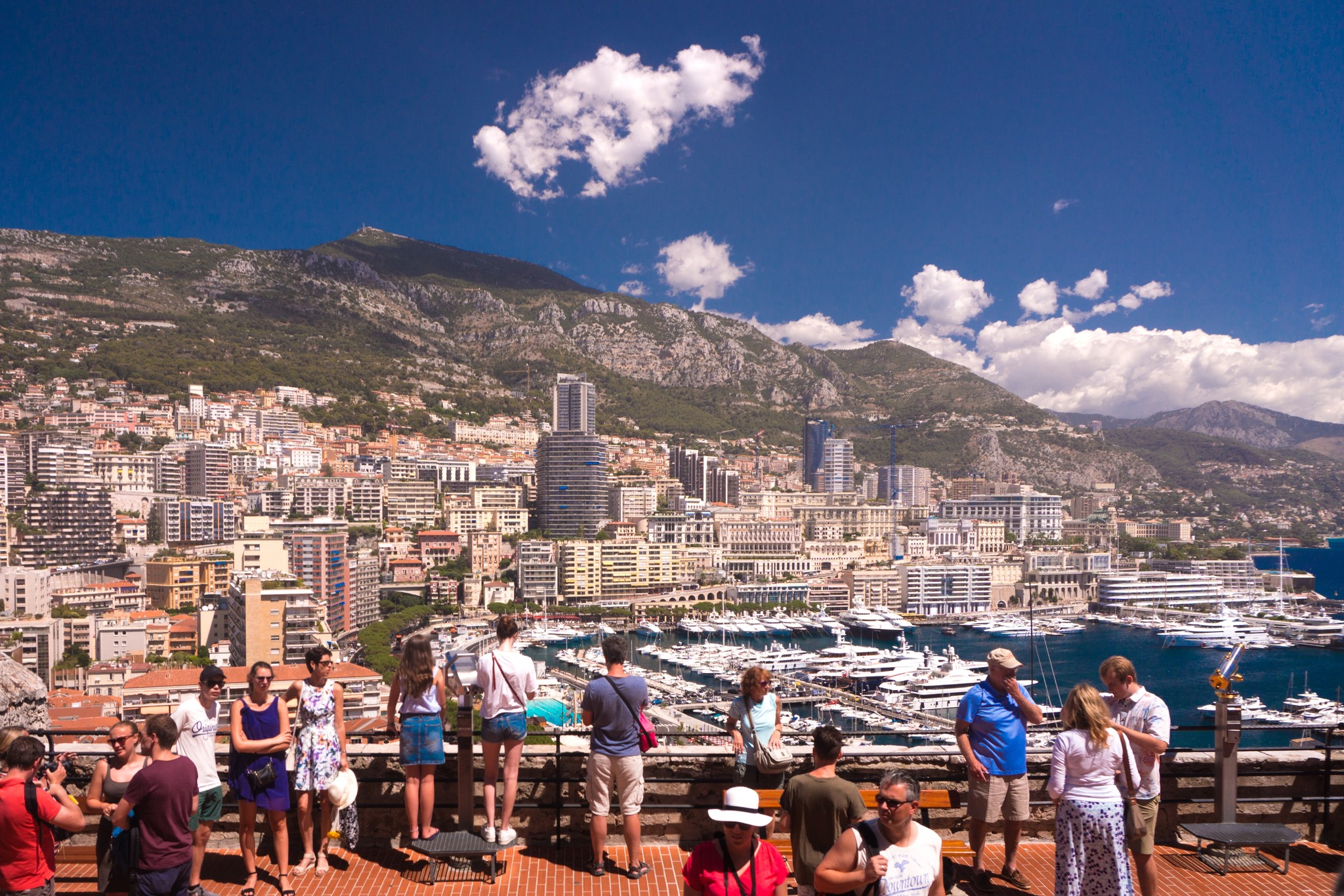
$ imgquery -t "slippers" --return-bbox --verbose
[410,825,440,841]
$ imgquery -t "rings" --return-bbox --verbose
[881,865,884,870]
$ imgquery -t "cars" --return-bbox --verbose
[661,591,669,595]
[1048,612,1054,615]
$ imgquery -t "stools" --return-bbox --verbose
[410,829,520,885]
[1179,822,1302,874]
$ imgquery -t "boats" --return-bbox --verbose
[481,603,1039,748]
[958,612,1087,637]
[1197,670,1344,737]
[1079,536,1344,652]
[941,627,956,635]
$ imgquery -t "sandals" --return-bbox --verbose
[628,861,649,880]
[585,859,605,876]
[240,851,328,896]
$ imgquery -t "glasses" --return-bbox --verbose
[107,735,134,746]
[875,794,914,808]
[758,680,772,687]
[202,680,225,687]
[319,660,333,666]
[253,677,274,682]
[724,821,752,830]
[993,667,1019,674]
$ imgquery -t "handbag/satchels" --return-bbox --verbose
[248,762,275,796]
[639,712,659,752]
[753,730,792,774]
[285,747,296,771]
[1125,799,1147,842]
[339,803,358,851]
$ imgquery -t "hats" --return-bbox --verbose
[987,648,1023,669]
[200,666,227,681]
[708,787,773,826]
[327,767,358,839]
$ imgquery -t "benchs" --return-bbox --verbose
[54,795,105,864]
[722,788,977,858]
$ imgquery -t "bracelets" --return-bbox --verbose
[340,750,347,753]
[776,729,782,736]
[387,721,395,726]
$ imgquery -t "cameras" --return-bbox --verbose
[39,758,74,777]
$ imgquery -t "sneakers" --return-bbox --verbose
[481,821,518,845]
[186,885,220,896]
[970,870,993,892]
[1002,866,1031,890]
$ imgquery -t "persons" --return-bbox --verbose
[726,666,782,840]
[1049,683,1140,896]
[681,725,947,896]
[169,665,227,896]
[0,714,199,896]
[226,662,296,896]
[279,646,349,876]
[386,634,446,841]
[579,634,651,879]
[476,615,538,845]
[954,647,1043,892]
[1099,655,1170,896]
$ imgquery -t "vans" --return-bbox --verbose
[1054,611,1060,614]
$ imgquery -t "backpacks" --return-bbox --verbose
[24,782,76,841]
